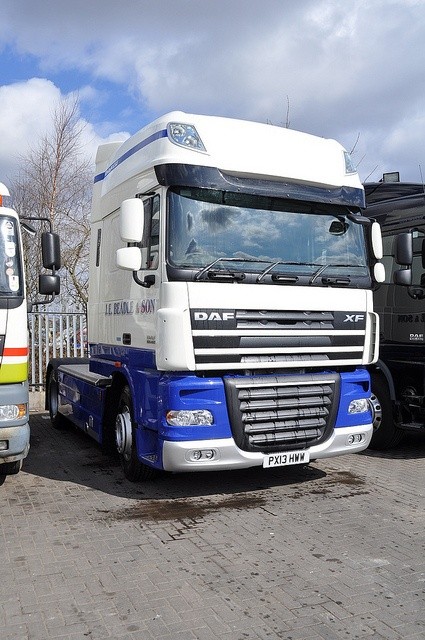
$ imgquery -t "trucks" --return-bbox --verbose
[0,181,61,476]
[39,110,387,485]
[362,170,425,454]
